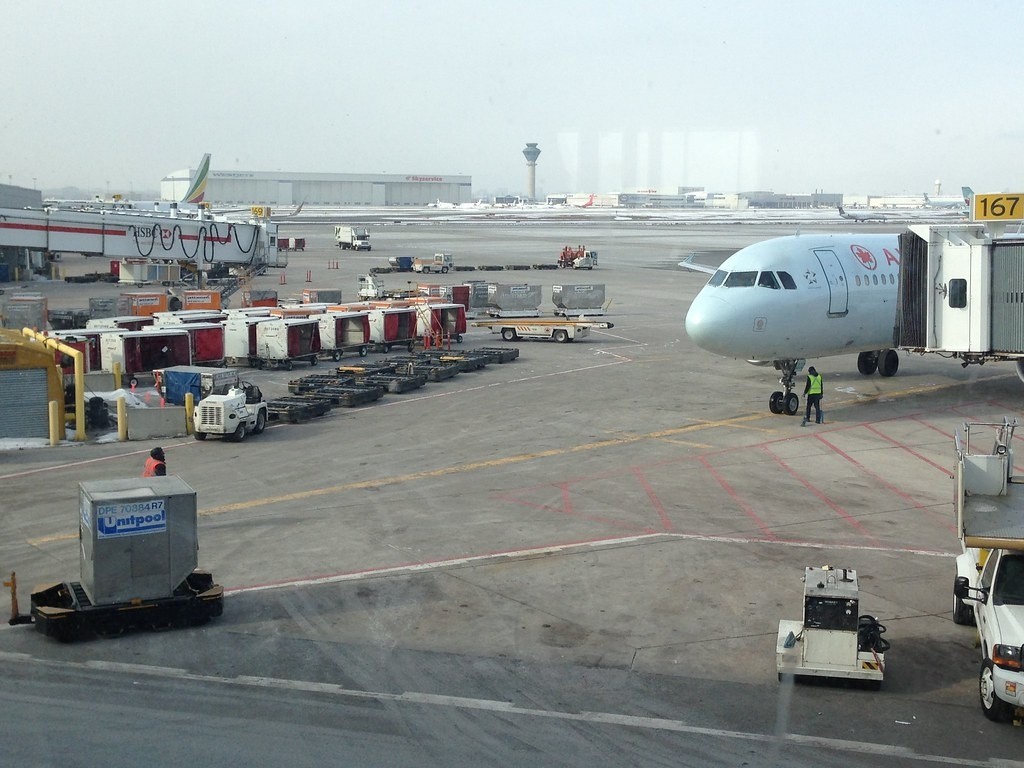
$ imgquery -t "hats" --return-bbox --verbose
[150,447,162,457]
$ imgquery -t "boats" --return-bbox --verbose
[427,191,617,222]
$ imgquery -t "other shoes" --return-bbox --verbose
[804,417,810,422]
[814,417,820,423]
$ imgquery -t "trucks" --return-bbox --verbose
[414,253,453,274]
[114,259,185,289]
[276,235,306,251]
[0,281,617,396]
[952,415,1024,728]
[557,245,600,270]
[156,367,242,406]
[335,225,372,251]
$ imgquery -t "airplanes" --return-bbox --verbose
[676,230,905,416]
[167,151,305,221]
[836,205,888,224]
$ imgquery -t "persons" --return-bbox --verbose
[142,447,166,477]
[801,366,824,425]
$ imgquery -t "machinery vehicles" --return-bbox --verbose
[193,379,270,442]
[775,564,891,691]
